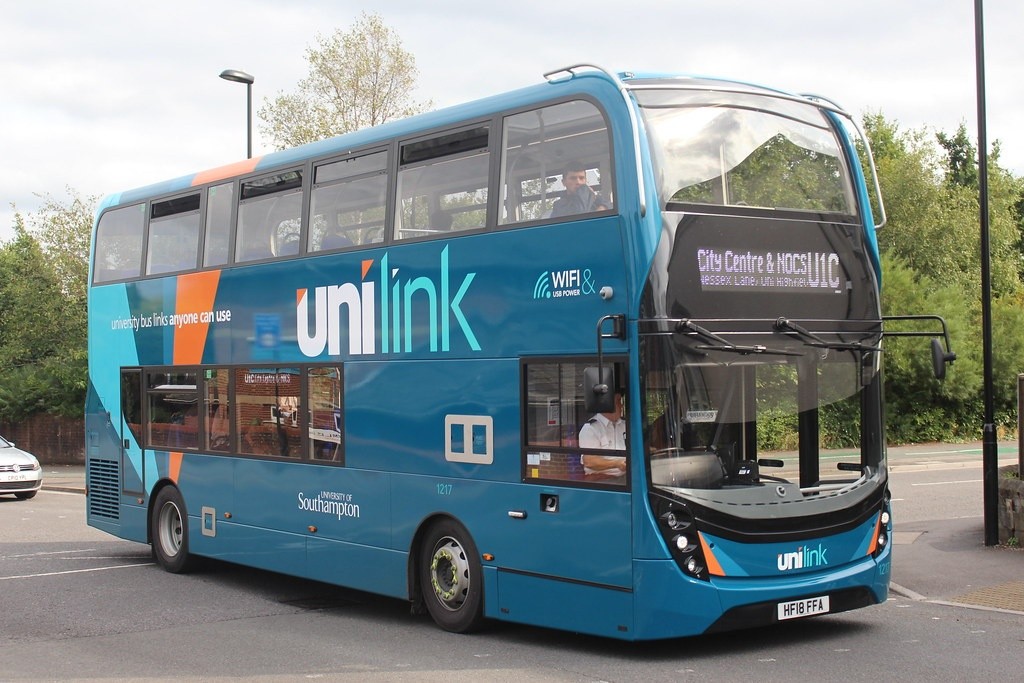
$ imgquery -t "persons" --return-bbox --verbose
[429,212,453,233]
[578,388,626,483]
[548,161,609,219]
[650,385,703,452]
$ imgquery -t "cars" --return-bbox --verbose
[0,436,44,500]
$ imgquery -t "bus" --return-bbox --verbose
[83,59,958,643]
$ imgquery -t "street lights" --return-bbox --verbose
[217,70,254,159]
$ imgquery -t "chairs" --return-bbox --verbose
[426,212,454,236]
[280,232,301,255]
[321,227,354,250]
[363,226,379,245]
[123,246,274,278]
